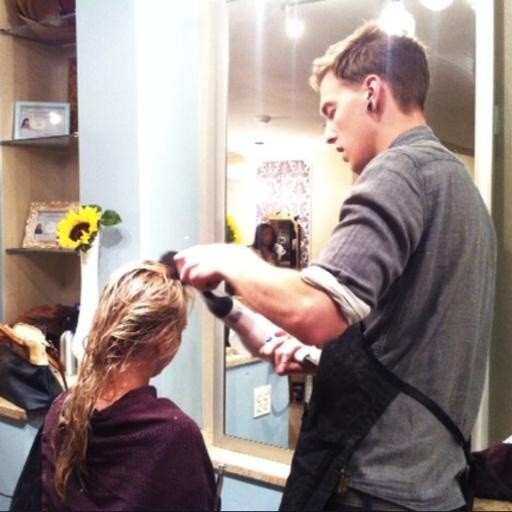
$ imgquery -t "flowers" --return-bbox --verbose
[225,215,242,245]
[55,205,123,254]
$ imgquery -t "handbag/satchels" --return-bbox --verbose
[1,348,62,412]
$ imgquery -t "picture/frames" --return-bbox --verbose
[13,100,71,146]
[21,200,78,250]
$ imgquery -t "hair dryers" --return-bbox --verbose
[205,286,308,362]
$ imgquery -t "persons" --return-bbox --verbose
[9,262,221,511]
[172,23,495,512]
[35,223,43,234]
[273,221,297,266]
[19,118,34,136]
[253,223,277,262]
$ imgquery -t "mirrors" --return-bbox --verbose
[199,0,497,490]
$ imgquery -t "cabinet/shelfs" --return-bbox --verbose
[1,13,79,257]
